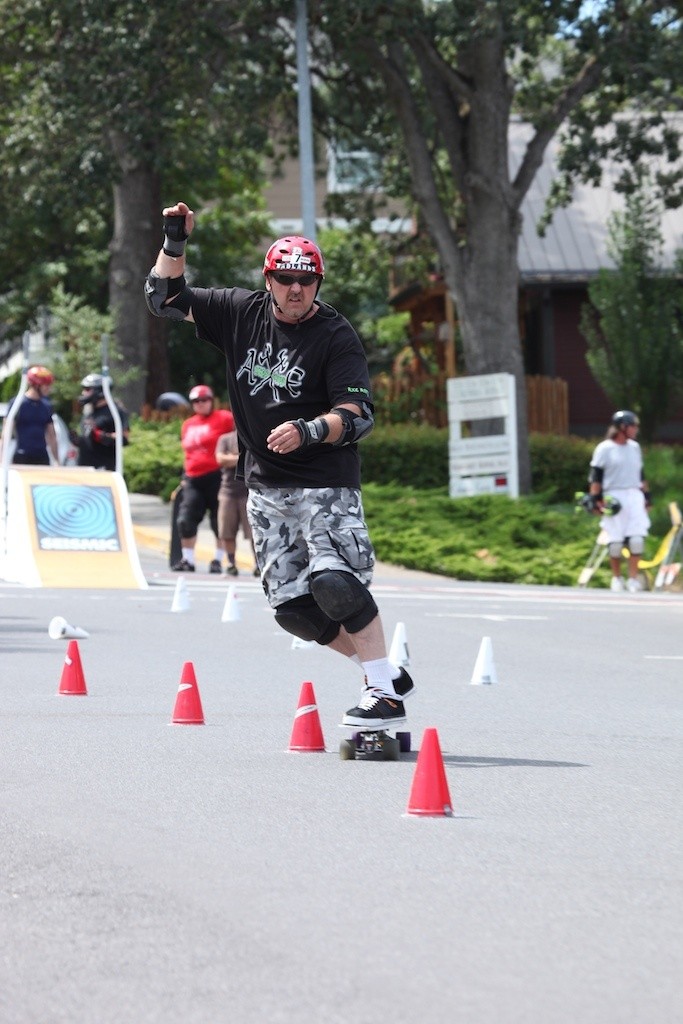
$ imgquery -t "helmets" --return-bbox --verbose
[28,366,53,385]
[612,411,640,426]
[81,374,114,389]
[189,385,213,400]
[262,236,324,278]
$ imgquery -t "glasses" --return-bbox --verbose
[83,387,92,391]
[268,271,319,286]
[193,398,209,403]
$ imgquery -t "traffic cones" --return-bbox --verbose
[48,619,94,643]
[166,573,190,621]
[53,637,92,699]
[289,681,331,752]
[407,727,459,815]
[217,580,243,628]
[387,621,414,668]
[466,635,502,692]
[170,661,210,726]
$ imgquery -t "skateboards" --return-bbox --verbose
[337,720,411,759]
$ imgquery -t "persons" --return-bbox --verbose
[69,374,129,471]
[171,385,266,575]
[589,410,652,592]
[144,203,416,724]
[0,367,59,467]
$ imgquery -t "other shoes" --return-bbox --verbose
[254,567,261,577]
[627,578,638,592]
[210,560,221,573]
[169,557,195,571]
[611,576,622,591]
[226,565,238,576]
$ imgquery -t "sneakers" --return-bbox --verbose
[343,687,407,727]
[361,666,415,698]
